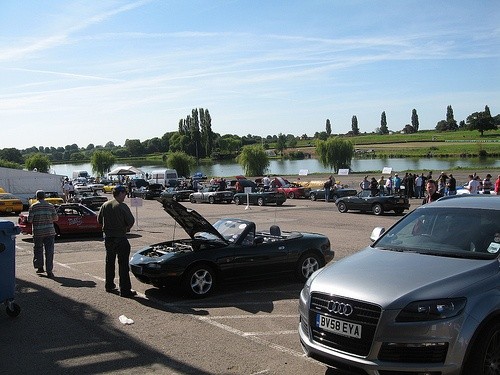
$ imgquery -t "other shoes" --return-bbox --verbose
[36,267,44,273]
[47,273,54,277]
[121,290,136,297]
[106,284,116,292]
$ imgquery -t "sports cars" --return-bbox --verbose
[129,195,334,298]
[233,179,286,206]
[160,187,194,203]
[273,177,305,199]
[335,188,410,216]
[309,188,357,201]
[189,187,234,204]
[67,191,108,210]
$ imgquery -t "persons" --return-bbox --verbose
[117,173,152,184]
[360,176,370,190]
[495,175,500,193]
[27,190,58,277]
[370,177,378,190]
[378,176,386,192]
[261,174,270,188]
[386,175,392,191]
[463,173,482,195]
[63,177,74,199]
[400,171,432,199]
[96,185,136,296]
[324,175,343,203]
[181,177,227,192]
[422,179,442,205]
[482,174,492,194]
[435,172,457,196]
[392,173,401,192]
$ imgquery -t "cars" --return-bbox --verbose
[377,179,405,193]
[0,188,23,216]
[103,182,128,193]
[26,191,64,207]
[62,171,107,195]
[298,194,500,375]
[127,169,264,200]
[17,202,106,239]
[354,147,374,153]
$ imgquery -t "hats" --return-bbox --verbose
[65,178,69,180]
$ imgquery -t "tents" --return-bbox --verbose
[108,166,145,182]
